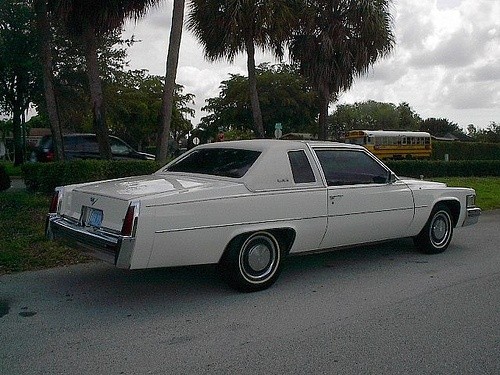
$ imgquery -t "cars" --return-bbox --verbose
[45,139,482,293]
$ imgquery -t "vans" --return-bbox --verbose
[29,134,155,163]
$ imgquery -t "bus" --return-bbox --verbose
[345,130,432,160]
